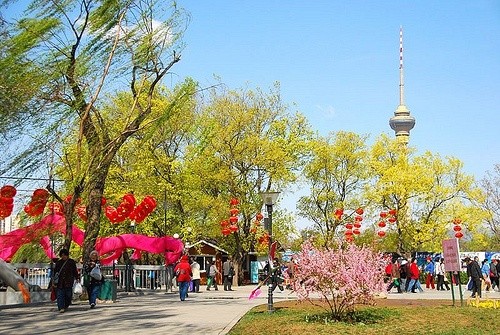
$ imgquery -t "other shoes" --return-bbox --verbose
[181,298,184,301]
[59,309,65,313]
[185,293,188,297]
[90,303,95,309]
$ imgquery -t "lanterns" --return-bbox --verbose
[220,197,239,236]
[335,207,363,242]
[104,193,157,225]
[48,194,107,223]
[378,210,397,237]
[0,185,17,220]
[453,218,463,238]
[251,212,264,234]
[24,189,49,217]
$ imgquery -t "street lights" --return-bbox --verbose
[258,191,281,312]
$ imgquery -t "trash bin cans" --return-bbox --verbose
[99,280,117,300]
[136,276,141,287]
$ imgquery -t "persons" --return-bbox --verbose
[223,258,233,291]
[174,255,191,301]
[206,261,220,291]
[53,250,78,313]
[148,258,211,293]
[84,250,104,308]
[383,257,500,298]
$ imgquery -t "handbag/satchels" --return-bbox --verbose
[190,280,193,292]
[52,272,59,290]
